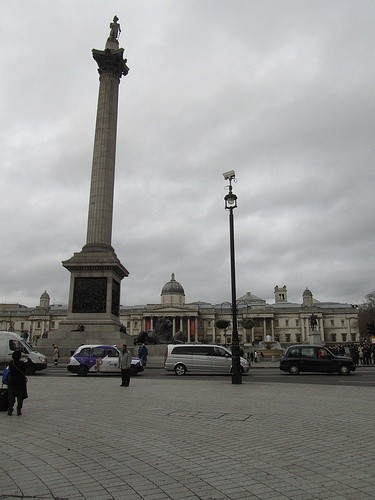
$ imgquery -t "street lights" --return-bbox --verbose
[222,170,244,385]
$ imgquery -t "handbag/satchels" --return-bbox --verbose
[25,377,29,383]
[1,368,10,385]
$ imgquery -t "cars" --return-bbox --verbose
[66,344,144,377]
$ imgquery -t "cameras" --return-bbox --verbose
[22,355,25,358]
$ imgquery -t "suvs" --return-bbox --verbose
[279,344,356,376]
[162,344,250,377]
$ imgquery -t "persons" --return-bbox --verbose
[23,331,28,341]
[246,352,253,361]
[349,337,375,365]
[310,313,316,328]
[260,352,264,357]
[138,344,148,366]
[71,324,84,332]
[119,344,131,387]
[254,351,258,362]
[138,331,147,343]
[52,344,59,366]
[329,343,345,356]
[7,351,28,416]
[42,329,48,338]
[120,326,126,333]
[110,16,121,39]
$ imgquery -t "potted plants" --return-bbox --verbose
[241,319,255,329]
[215,320,230,329]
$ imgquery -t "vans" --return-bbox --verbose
[0,331,48,377]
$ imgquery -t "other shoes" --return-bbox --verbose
[120,384,128,387]
[17,413,22,416]
[7,413,12,416]
